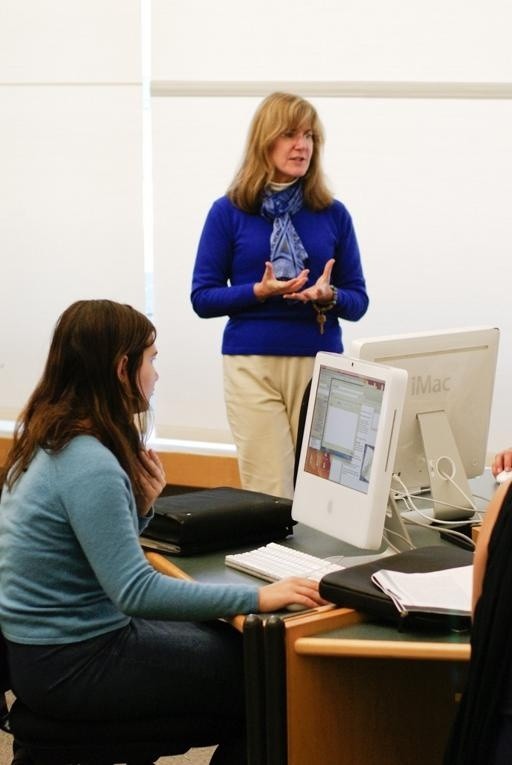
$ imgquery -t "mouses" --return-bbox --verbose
[496,468,511,484]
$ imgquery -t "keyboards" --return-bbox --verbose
[223,542,347,591]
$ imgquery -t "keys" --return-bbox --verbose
[317,313,327,335]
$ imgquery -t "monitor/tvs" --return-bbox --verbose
[291,351,417,569]
[355,326,502,528]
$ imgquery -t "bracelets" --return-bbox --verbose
[312,285,338,313]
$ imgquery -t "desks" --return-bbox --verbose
[145,521,445,765]
[260,608,472,765]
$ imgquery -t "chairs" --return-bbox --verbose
[0,628,224,764]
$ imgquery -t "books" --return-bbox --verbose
[138,526,298,556]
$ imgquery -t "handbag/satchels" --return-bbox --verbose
[317,547,474,631]
[136,486,293,555]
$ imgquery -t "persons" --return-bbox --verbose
[189,92,369,499]
[0,300,329,765]
